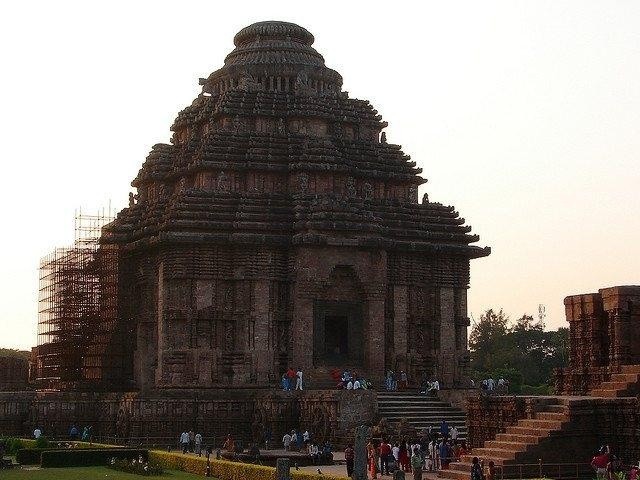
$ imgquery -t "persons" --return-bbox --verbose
[221,362,510,479]
[89,424,95,445]
[33,425,42,439]
[179,429,191,454]
[629,464,640,480]
[188,427,195,452]
[68,424,80,441]
[611,460,627,480]
[194,431,203,454]
[82,426,89,442]
[591,443,610,480]
